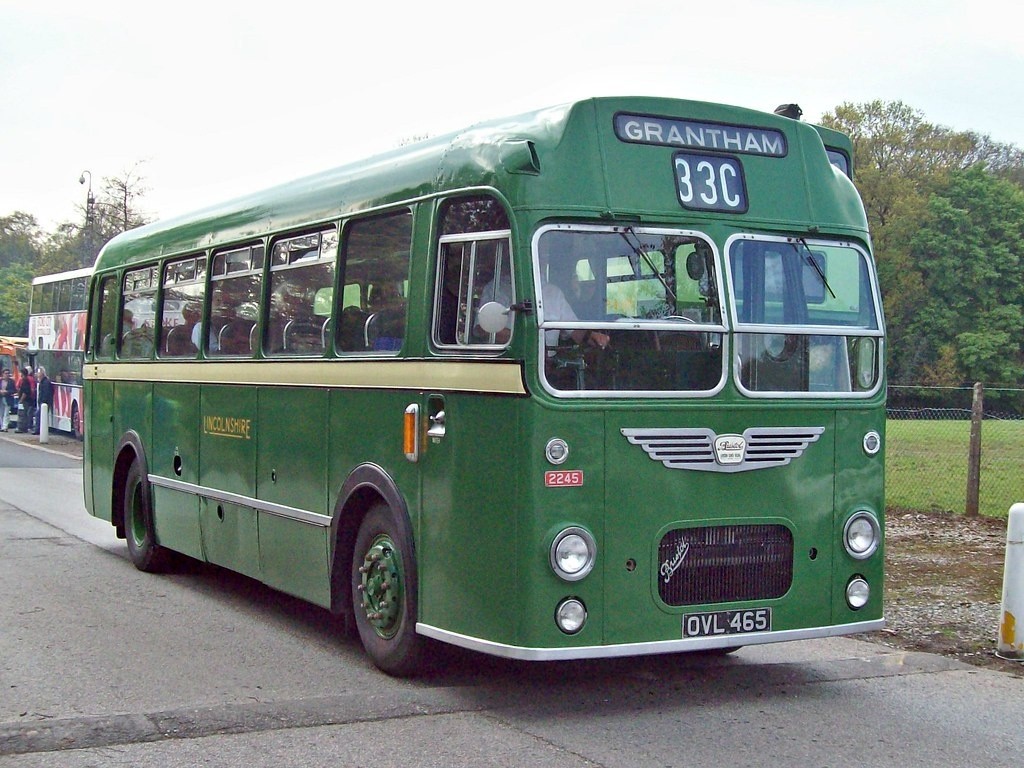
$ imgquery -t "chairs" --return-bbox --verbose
[99,310,404,357]
[656,315,703,351]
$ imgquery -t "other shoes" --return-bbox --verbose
[31,432,40,435]
[0,429,8,432]
[15,430,24,433]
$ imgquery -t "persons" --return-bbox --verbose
[440,239,610,368]
[103,286,406,359]
[0,365,54,435]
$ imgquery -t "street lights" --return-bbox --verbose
[79,170,96,228]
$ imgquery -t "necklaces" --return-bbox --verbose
[56,369,78,384]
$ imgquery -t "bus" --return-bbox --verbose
[10,350,21,357]
[0,336,28,428]
[28,267,95,440]
[83,96,886,675]
